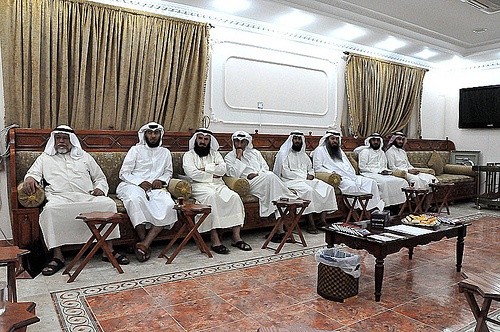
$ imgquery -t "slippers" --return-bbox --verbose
[231,239,252,251]
[211,244,229,254]
[102,249,130,265]
[42,258,65,276]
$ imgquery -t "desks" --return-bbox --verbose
[428,182,455,215]
[342,191,374,223]
[0,300,41,332]
[321,213,473,302]
[156,203,213,264]
[0,245,31,302]
[61,210,123,283]
[261,198,312,254]
[398,186,430,215]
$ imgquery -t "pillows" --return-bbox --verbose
[427,150,444,176]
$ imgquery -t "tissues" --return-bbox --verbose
[370,199,391,229]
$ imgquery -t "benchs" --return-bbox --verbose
[5,125,484,280]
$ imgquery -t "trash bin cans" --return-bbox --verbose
[317,248,360,302]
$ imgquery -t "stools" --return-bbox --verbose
[458,269,500,332]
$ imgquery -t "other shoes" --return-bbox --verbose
[134,240,152,263]
[317,222,328,231]
[265,232,295,243]
[305,225,319,234]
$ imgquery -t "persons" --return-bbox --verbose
[225,130,298,238]
[116,122,178,262]
[352,133,411,207]
[23,124,130,276]
[182,128,252,253]
[273,130,338,235]
[385,131,438,213]
[310,130,385,212]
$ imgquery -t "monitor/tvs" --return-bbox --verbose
[457,85,500,129]
